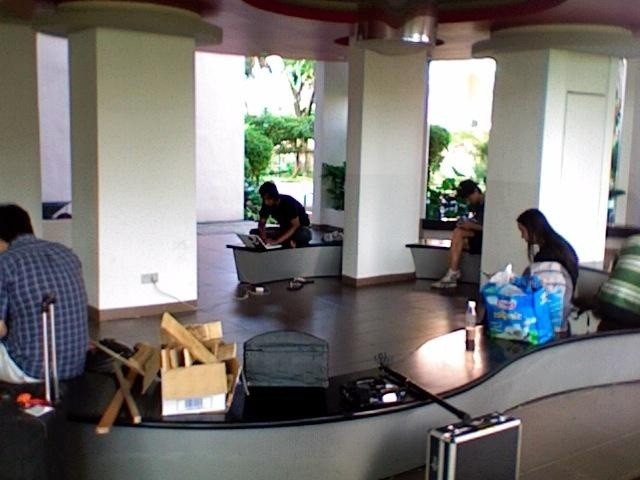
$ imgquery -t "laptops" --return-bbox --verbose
[235,232,283,250]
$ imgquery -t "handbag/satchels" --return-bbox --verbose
[482,272,556,346]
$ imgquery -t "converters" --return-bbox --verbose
[255,286,264,292]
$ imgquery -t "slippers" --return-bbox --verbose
[286,275,316,291]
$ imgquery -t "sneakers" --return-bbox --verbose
[431,267,463,289]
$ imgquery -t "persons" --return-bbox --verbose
[430,179,485,289]
[480,208,579,324]
[249,181,313,247]
[0,203,91,385]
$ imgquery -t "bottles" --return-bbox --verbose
[464,301,477,340]
[465,342,475,375]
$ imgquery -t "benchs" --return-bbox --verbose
[575,261,614,300]
[406,239,479,283]
[226,232,343,285]
[0,326,640,480]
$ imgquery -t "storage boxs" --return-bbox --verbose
[161,358,242,417]
[244,329,330,390]
